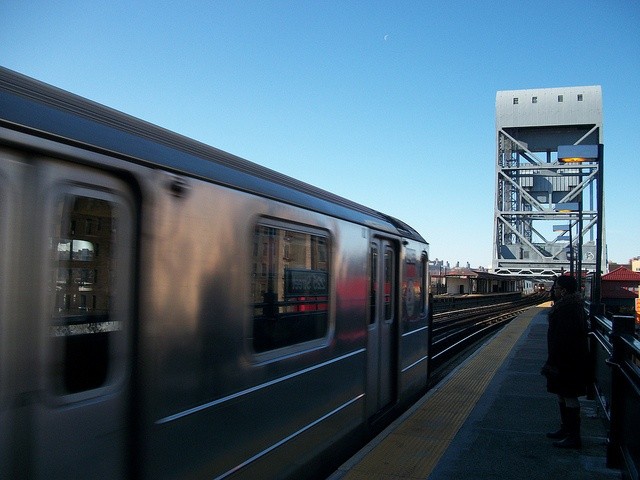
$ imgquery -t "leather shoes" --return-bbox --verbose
[547,430,566,439]
[553,436,580,449]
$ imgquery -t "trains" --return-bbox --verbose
[3,65,435,479]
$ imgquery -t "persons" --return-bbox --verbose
[541,275,592,449]
[580,284,585,298]
[550,279,558,305]
